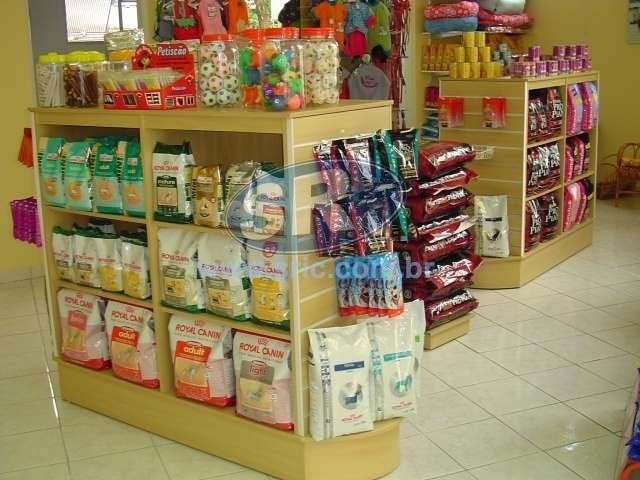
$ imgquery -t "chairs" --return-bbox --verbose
[603,143,640,203]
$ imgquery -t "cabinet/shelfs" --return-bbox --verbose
[411,0,598,297]
[26,99,405,480]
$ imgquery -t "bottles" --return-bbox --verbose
[198,32,240,108]
[579,44,588,58]
[36,52,66,108]
[552,45,565,59]
[262,27,304,111]
[469,62,480,79]
[479,46,489,61]
[464,46,477,62]
[78,52,105,108]
[63,50,80,108]
[509,58,592,78]
[454,46,463,62]
[576,45,588,59]
[458,62,469,79]
[104,30,144,61]
[480,61,493,77]
[449,62,458,79]
[239,29,262,112]
[529,45,541,62]
[473,30,485,48]
[301,28,339,107]
[462,32,474,46]
